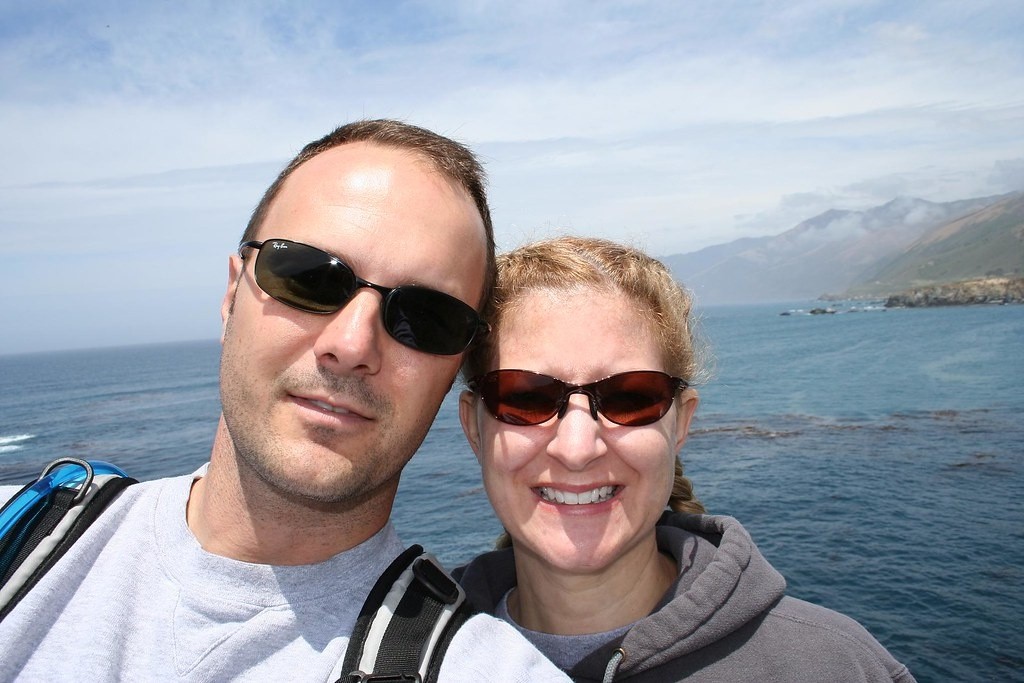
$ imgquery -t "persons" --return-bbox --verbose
[2,119,574,683]
[454,238,915,683]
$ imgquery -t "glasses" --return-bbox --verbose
[468,369,688,426]
[237,238,493,355]
